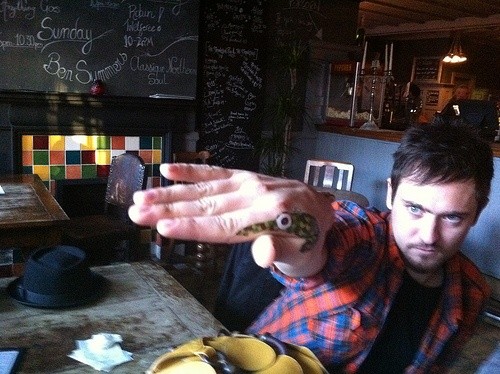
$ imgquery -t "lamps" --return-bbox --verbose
[442,31,467,64]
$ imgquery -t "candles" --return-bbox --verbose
[384,44,388,70]
[361,41,367,70]
[389,43,393,70]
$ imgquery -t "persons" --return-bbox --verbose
[451,83,483,126]
[128,122,494,374]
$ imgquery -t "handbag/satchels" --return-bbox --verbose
[145,331,329,374]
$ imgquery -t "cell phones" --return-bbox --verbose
[0,346,24,374]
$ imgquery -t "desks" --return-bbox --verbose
[213,185,369,274]
[0,173,72,275]
[0,258,235,374]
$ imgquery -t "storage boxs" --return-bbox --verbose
[411,56,455,84]
[416,85,454,111]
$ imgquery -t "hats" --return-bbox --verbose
[7,244,103,310]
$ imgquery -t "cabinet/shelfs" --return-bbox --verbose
[315,122,500,329]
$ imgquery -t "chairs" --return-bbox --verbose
[304,159,355,192]
[168,150,211,259]
[61,152,147,262]
